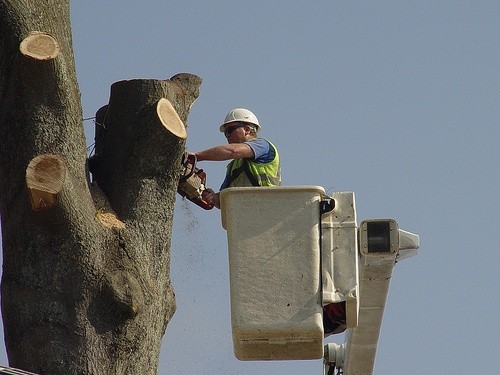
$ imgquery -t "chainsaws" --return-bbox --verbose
[177,151,217,212]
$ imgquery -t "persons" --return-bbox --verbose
[187,107,283,209]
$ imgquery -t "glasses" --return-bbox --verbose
[224,125,245,133]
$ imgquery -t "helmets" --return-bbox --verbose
[219,108,262,134]
